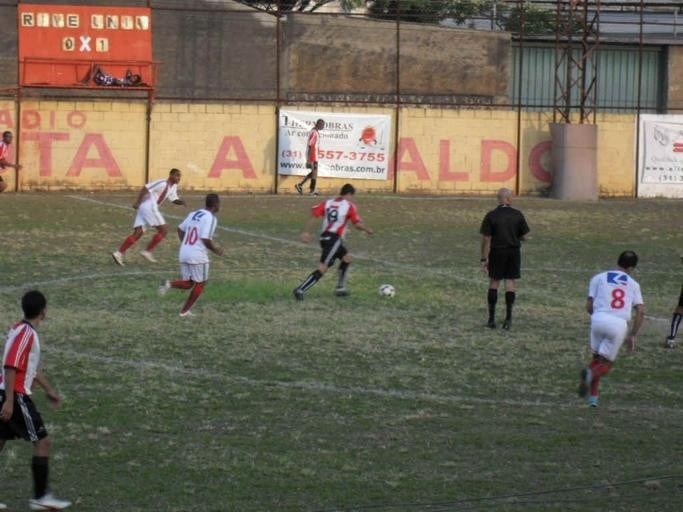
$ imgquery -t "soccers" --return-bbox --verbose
[378,283,396,300]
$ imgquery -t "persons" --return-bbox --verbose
[294,119,326,197]
[159,192,224,319]
[0,288,72,507]
[0,131,23,194]
[112,167,189,268]
[478,187,532,330]
[664,282,683,347]
[293,183,374,301]
[74,63,143,88]
[578,249,646,410]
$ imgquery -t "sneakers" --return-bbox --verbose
[140,250,157,264]
[112,251,125,267]
[294,184,303,194]
[488,320,497,329]
[293,288,304,301]
[158,278,171,297]
[503,318,510,330]
[334,286,352,296]
[589,395,599,407]
[28,492,72,511]
[308,190,319,196]
[179,310,192,316]
[578,367,590,401]
